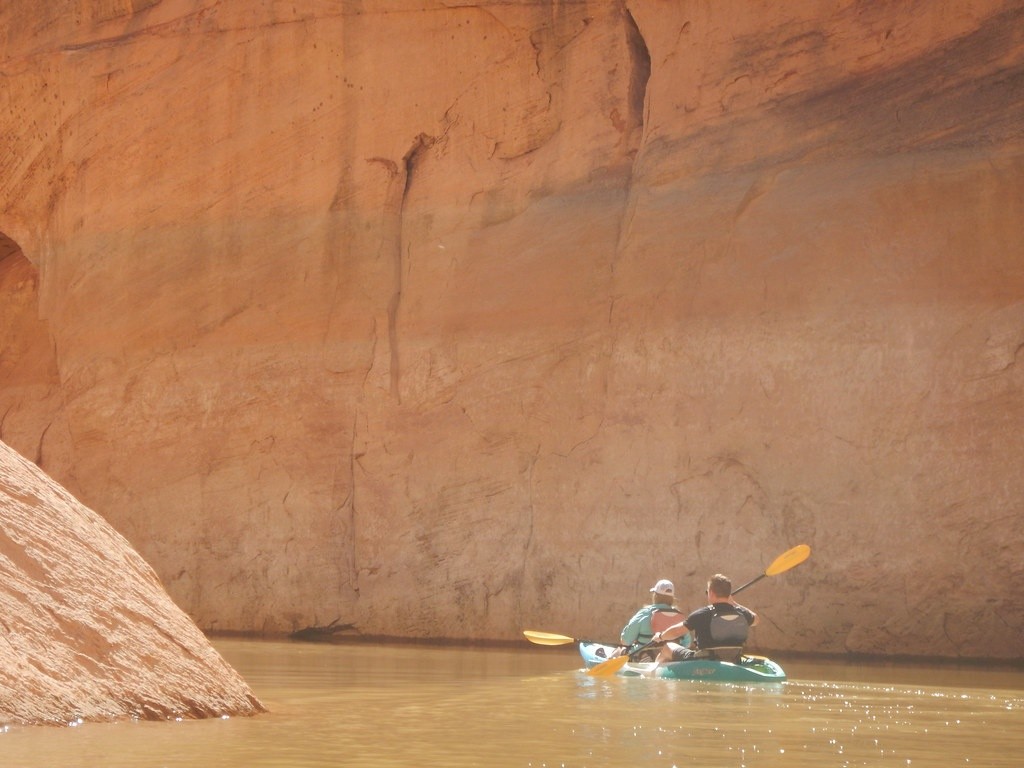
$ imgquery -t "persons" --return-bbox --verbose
[652,574,758,663]
[611,579,691,663]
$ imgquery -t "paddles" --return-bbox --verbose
[589,544,811,677]
[523,630,624,648]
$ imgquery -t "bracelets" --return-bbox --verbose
[659,634,663,640]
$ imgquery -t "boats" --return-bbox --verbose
[578,642,787,683]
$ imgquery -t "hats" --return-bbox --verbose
[649,580,674,596]
